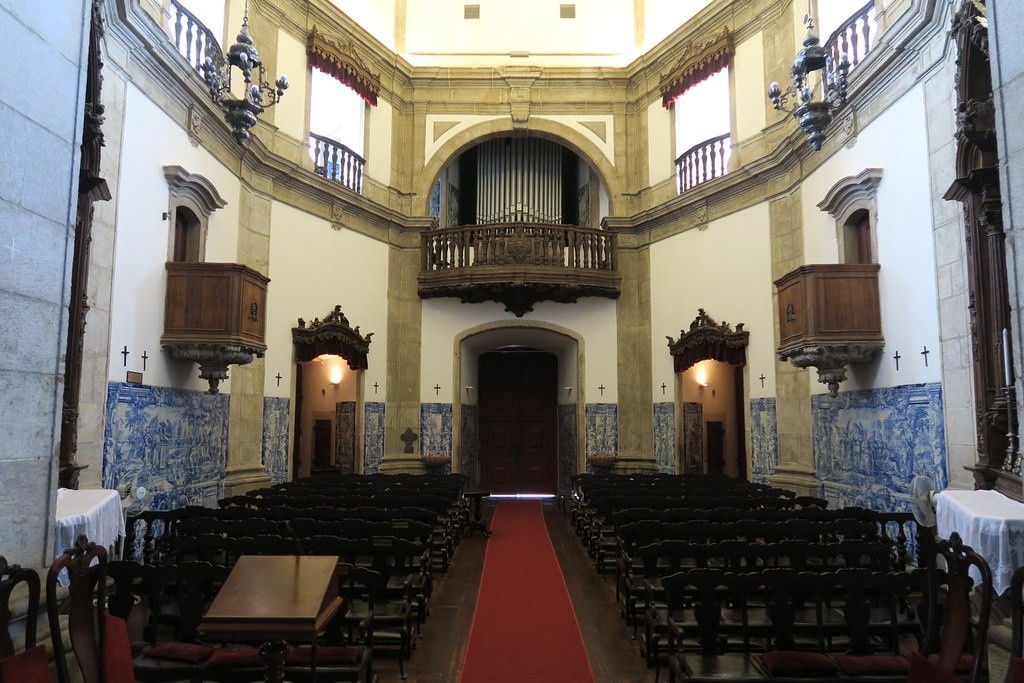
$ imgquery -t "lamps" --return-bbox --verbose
[199,0,289,145]
[765,0,852,151]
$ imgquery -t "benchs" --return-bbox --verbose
[556,469,1024,683]
[0,455,491,683]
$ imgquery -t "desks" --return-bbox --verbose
[54,488,126,588]
[936,489,1024,617]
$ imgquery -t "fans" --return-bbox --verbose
[908,475,940,527]
